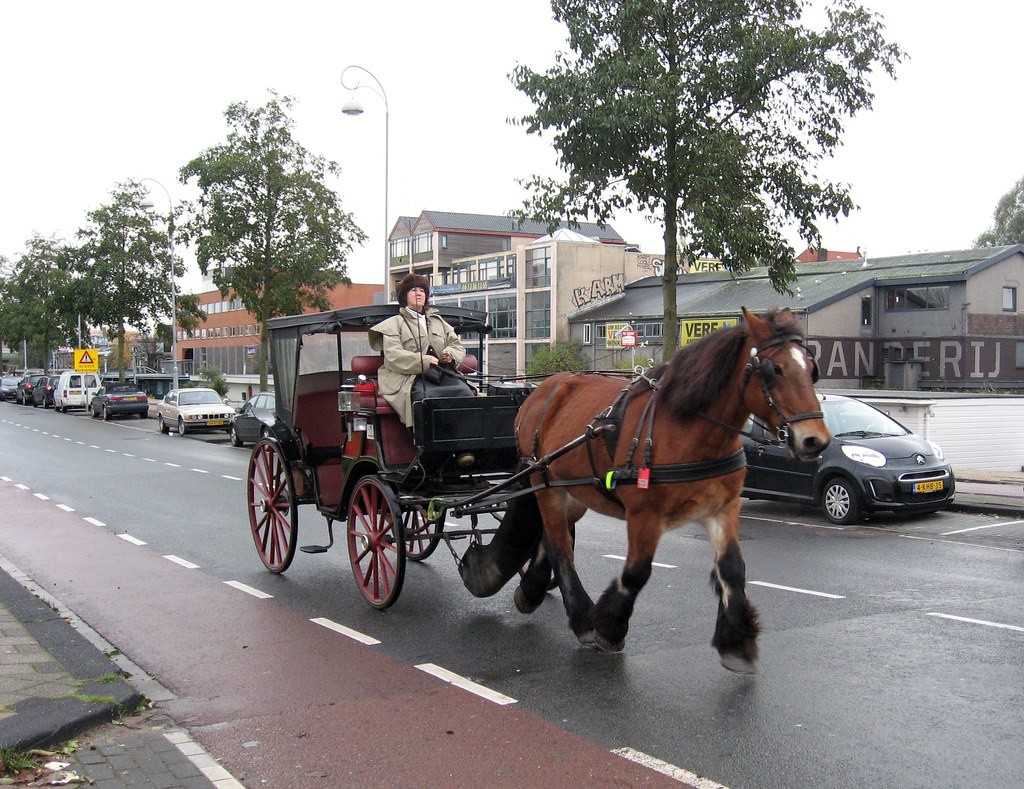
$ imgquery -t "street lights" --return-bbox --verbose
[137,178,179,389]
[340,65,391,306]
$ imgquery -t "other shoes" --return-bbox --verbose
[456,452,476,469]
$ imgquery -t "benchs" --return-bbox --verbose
[350,353,478,415]
[292,390,340,462]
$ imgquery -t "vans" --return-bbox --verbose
[53,372,102,413]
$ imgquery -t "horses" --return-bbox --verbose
[511,305,834,676]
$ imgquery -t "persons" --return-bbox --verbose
[367,274,478,467]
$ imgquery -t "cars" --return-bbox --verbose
[155,387,236,437]
[31,375,73,409]
[0,374,45,406]
[737,394,956,525]
[91,381,149,421]
[228,391,275,452]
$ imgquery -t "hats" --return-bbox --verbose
[395,273,429,308]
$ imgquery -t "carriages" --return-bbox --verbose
[247,304,831,675]
